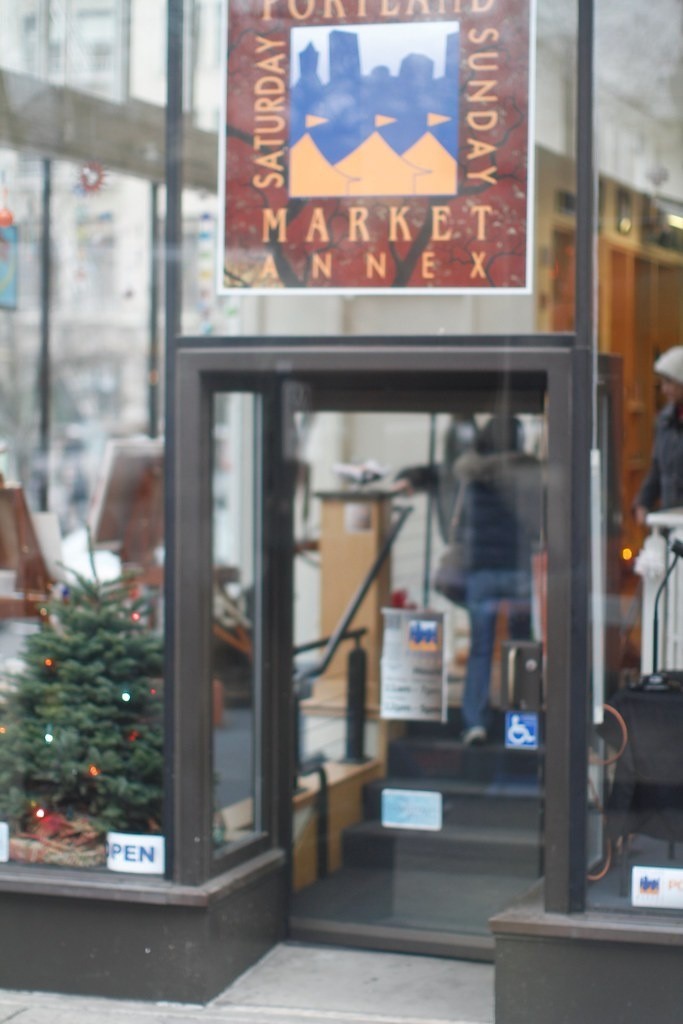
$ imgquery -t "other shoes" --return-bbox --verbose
[462,726,489,745]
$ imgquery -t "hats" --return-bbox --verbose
[653,343,683,383]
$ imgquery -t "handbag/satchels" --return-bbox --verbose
[433,542,467,607]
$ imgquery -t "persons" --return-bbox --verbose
[629,347,683,691]
[448,416,547,745]
